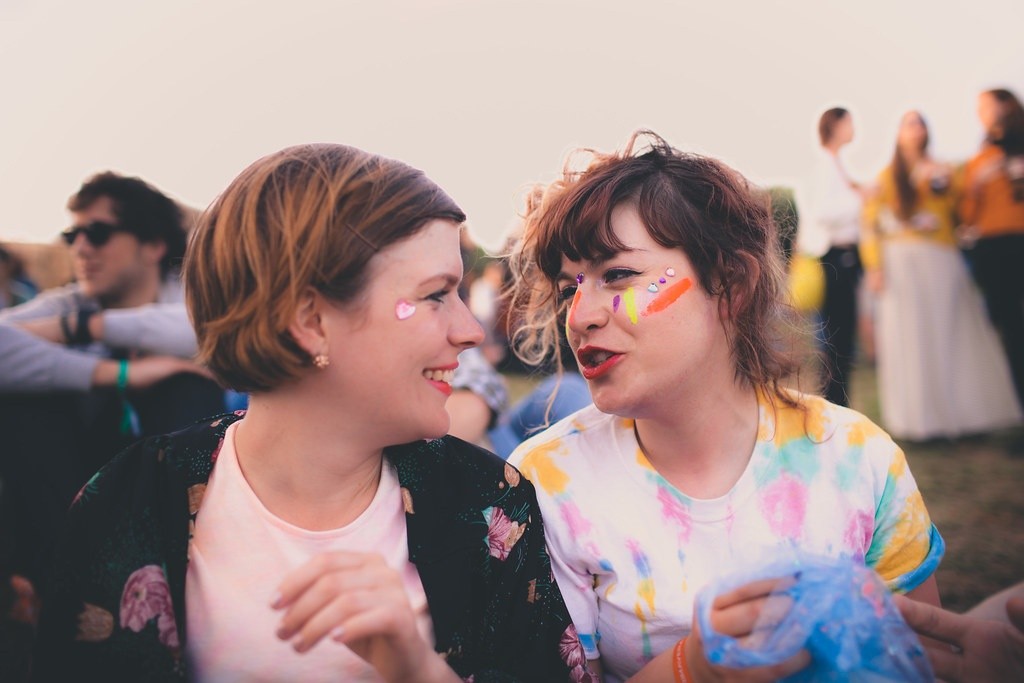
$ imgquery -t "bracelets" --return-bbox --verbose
[57,306,97,349]
[674,634,694,682]
[116,354,129,391]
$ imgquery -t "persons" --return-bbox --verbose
[859,112,1020,442]
[793,105,866,407]
[20,141,592,682]
[494,129,949,682]
[1,168,228,393]
[891,575,1022,679]
[443,348,506,448]
[950,90,1024,410]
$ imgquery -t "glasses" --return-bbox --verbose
[62,221,138,247]
